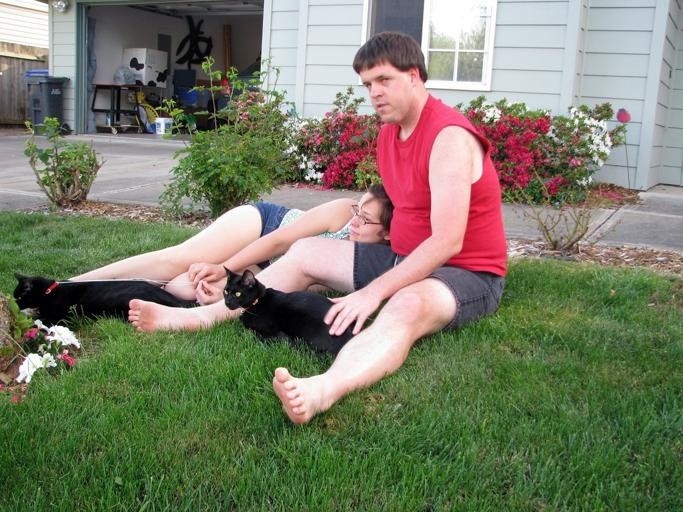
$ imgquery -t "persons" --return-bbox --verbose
[66,183,396,308]
[125,31,508,425]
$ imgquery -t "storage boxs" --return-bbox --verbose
[123,48,168,89]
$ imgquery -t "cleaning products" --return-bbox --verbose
[106,113,111,127]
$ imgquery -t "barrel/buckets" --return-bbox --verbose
[155,117,174,135]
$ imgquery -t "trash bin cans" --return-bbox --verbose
[23,70,70,136]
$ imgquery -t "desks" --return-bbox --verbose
[90,83,145,128]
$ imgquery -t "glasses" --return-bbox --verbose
[349,204,384,226]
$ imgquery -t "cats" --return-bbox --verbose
[223,265,364,355]
[13,274,180,328]
